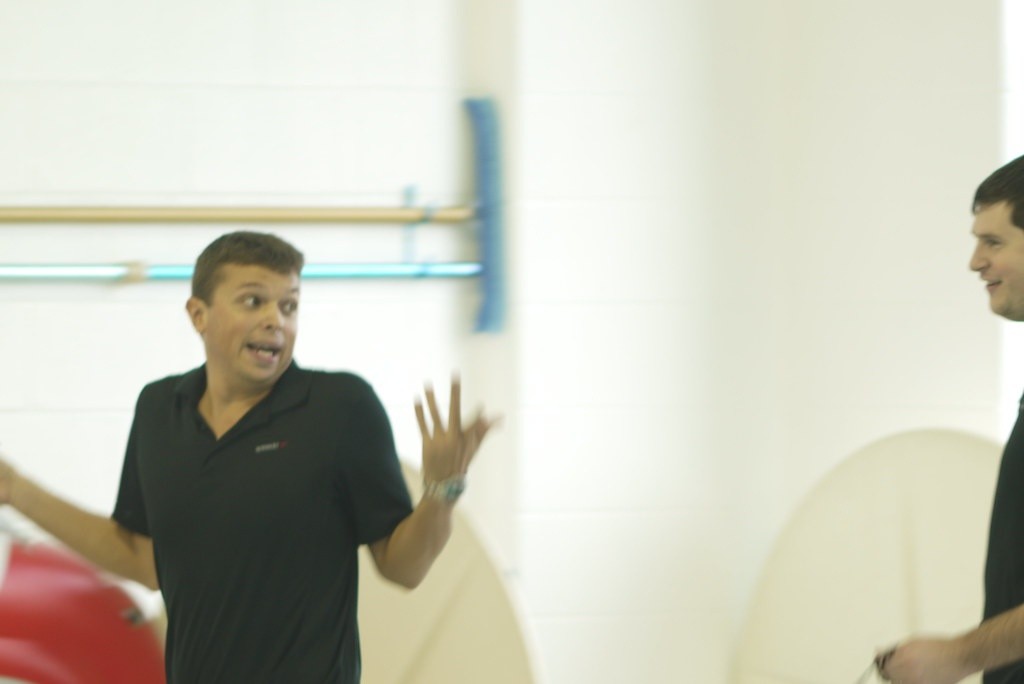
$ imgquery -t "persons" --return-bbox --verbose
[0,230,503,684]
[873,156,1024,684]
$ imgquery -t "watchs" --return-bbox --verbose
[423,474,465,505]
